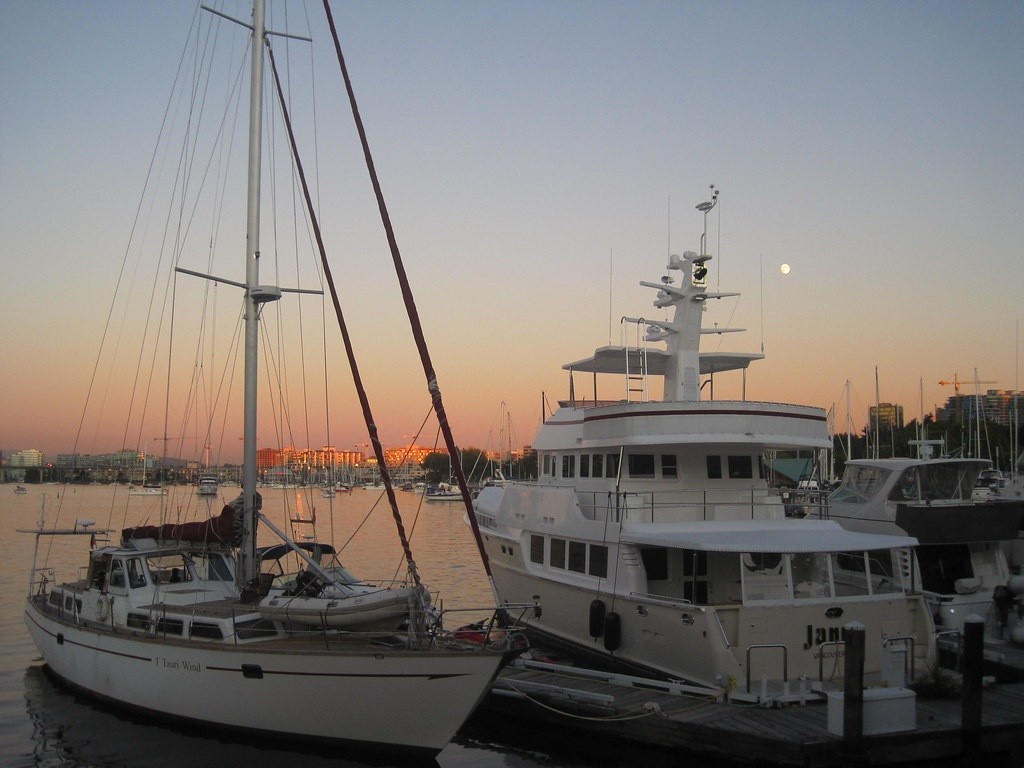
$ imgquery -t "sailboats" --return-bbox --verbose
[425,401,531,500]
[125,441,168,495]
[13,0,542,762]
[782,367,1024,673]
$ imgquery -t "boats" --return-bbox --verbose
[197,472,217,494]
[464,180,941,701]
[15,486,30,494]
[321,480,398,499]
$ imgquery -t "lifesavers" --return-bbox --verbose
[95,595,110,621]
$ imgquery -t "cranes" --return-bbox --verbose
[938,372,996,395]
